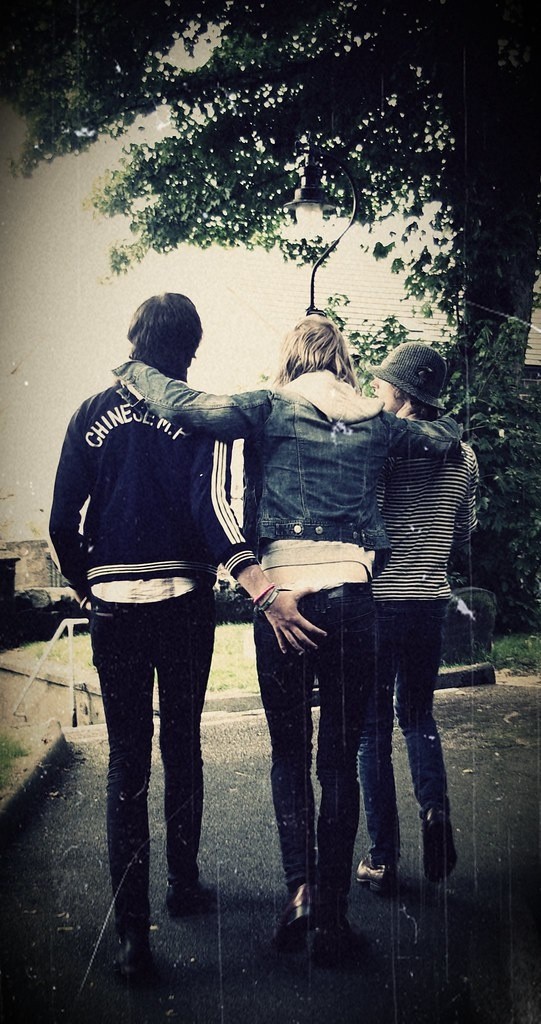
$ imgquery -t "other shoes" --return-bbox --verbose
[282,881,350,935]
[356,855,397,894]
[166,879,219,918]
[110,925,149,975]
[421,807,457,882]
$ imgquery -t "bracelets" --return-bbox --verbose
[254,584,279,611]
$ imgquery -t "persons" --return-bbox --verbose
[360,342,478,895]
[49,296,328,985]
[111,317,460,964]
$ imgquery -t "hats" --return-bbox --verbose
[367,342,447,411]
